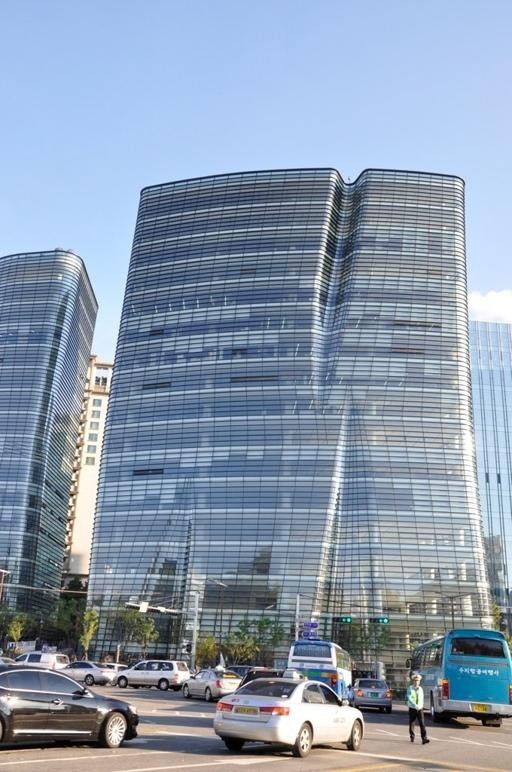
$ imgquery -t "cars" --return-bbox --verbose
[349,677,393,715]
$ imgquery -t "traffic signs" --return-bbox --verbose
[303,622,319,629]
[302,632,319,638]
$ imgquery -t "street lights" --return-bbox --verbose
[418,588,492,631]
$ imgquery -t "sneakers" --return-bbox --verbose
[411,737,429,744]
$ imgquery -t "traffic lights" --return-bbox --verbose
[331,617,352,623]
[369,617,390,624]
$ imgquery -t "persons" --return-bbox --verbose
[406,674,431,744]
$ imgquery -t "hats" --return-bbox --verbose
[411,675,422,681]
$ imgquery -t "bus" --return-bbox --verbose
[288,640,387,702]
[404,626,512,727]
